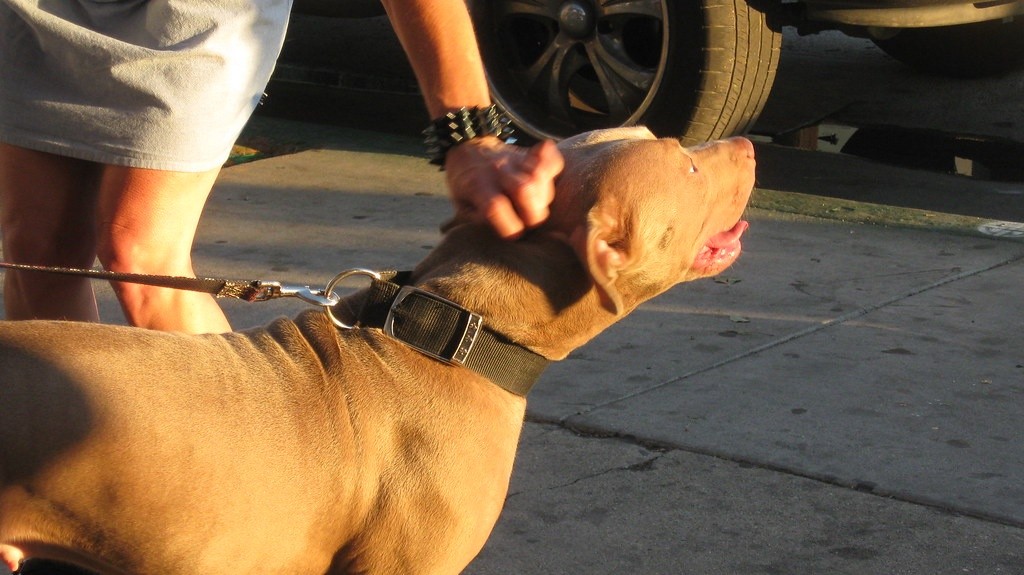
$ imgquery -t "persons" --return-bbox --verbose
[0,0,568,334]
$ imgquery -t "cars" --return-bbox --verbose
[469,0,1018,151]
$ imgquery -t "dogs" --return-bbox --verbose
[0,126,759,575]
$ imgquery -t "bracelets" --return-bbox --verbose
[421,103,519,172]
